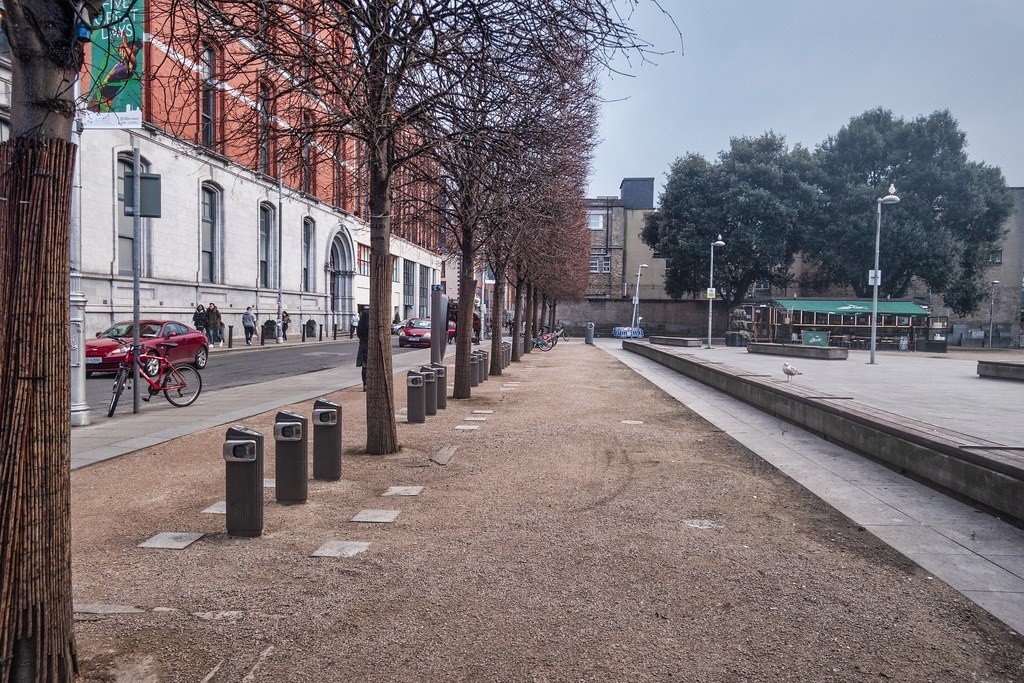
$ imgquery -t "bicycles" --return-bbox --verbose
[107,335,202,418]
[483,320,570,352]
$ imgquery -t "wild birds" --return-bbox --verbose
[783,363,803,381]
[718,234,722,241]
[888,184,896,195]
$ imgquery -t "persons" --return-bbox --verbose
[283,310,291,340]
[241,306,256,346]
[473,318,481,343]
[192,304,207,332]
[86,30,144,113]
[351,313,360,335]
[357,309,369,391]
[206,303,223,347]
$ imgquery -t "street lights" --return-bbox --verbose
[631,264,648,339]
[989,281,1000,347]
[870,195,900,363]
[708,241,725,348]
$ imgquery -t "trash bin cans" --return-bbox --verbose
[585,322,594,344]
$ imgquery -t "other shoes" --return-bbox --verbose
[248,340,252,346]
[363,385,367,392]
[219,340,223,346]
[209,344,213,347]
[284,338,288,343]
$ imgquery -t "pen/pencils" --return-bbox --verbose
[1020,329,1024,348]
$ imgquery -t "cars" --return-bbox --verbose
[391,318,409,335]
[399,317,456,348]
[85,319,209,378]
